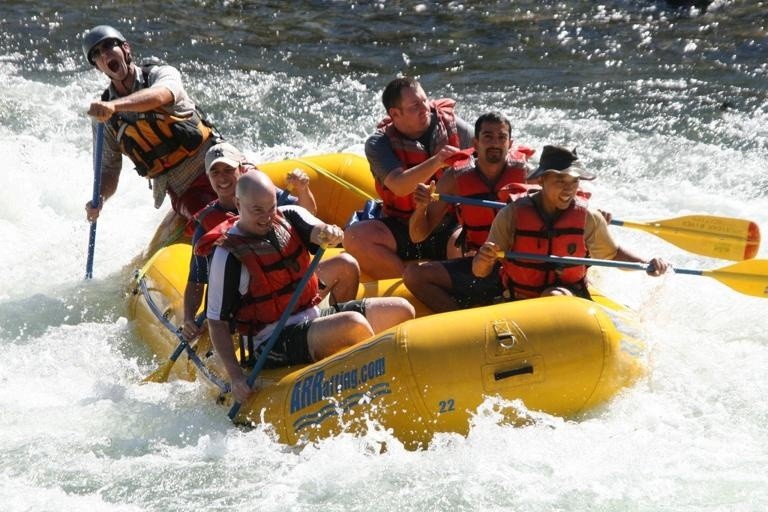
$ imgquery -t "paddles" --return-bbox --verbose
[494,250,768,298]
[429,193,759,261]
[140,182,295,384]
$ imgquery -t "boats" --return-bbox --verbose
[128,150,655,455]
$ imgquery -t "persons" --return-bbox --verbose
[81,25,247,222]
[342,76,475,281]
[204,170,416,405]
[179,141,362,344]
[471,143,673,303]
[402,110,611,314]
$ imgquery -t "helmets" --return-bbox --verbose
[82,24,126,65]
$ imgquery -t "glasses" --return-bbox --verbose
[90,39,120,62]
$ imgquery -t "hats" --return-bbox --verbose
[205,143,242,173]
[526,146,596,180]
